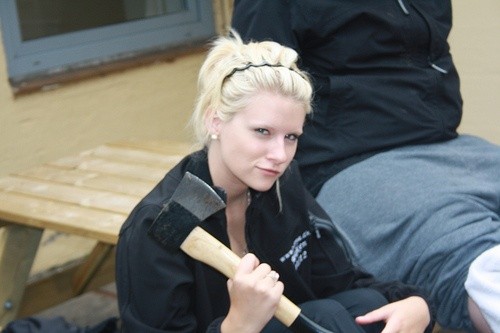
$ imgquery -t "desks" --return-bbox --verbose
[1,147,185,330]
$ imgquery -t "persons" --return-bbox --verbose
[224,0,500,333]
[113,27,437,333]
